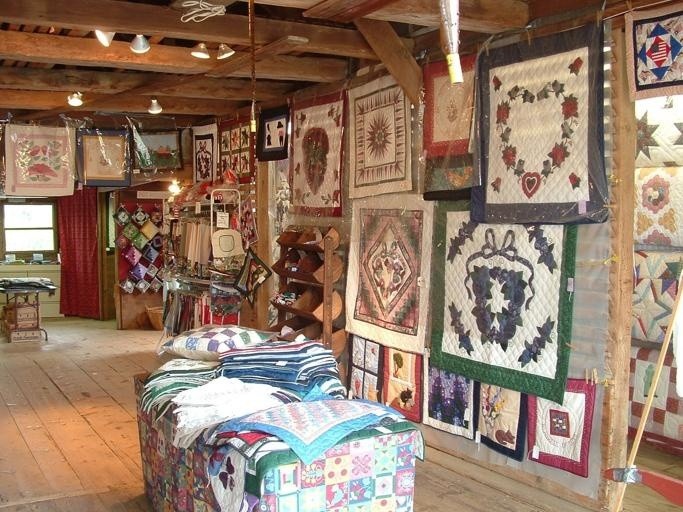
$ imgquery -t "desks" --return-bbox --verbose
[131,374,427,512]
[0,284,53,348]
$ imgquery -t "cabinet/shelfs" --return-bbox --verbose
[271,225,349,394]
[158,184,249,339]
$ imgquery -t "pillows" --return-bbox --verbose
[157,324,279,367]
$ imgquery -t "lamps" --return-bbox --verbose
[95,29,119,47]
[215,44,237,59]
[128,34,153,56]
[190,43,212,62]
[66,90,85,107]
[147,96,164,116]
